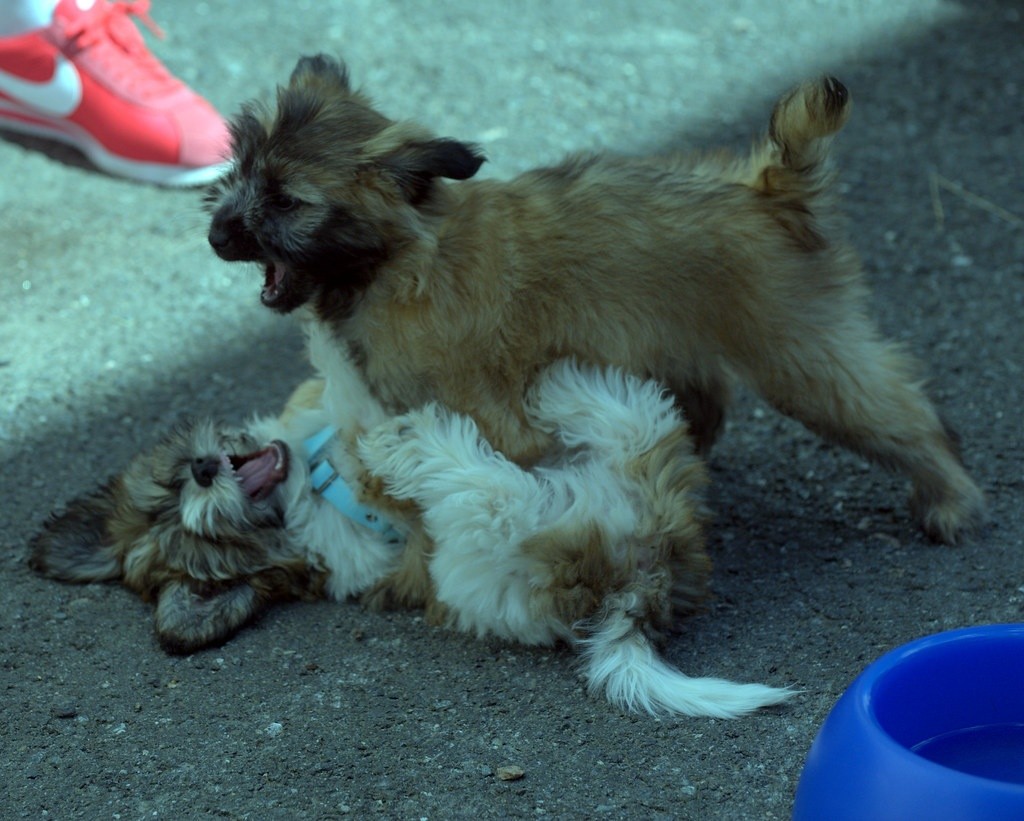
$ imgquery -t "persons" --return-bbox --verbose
[0,0,239,187]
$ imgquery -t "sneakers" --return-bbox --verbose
[0,0,240,188]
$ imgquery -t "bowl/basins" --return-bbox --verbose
[791,622,1024,821]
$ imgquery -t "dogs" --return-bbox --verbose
[28,46,996,724]
